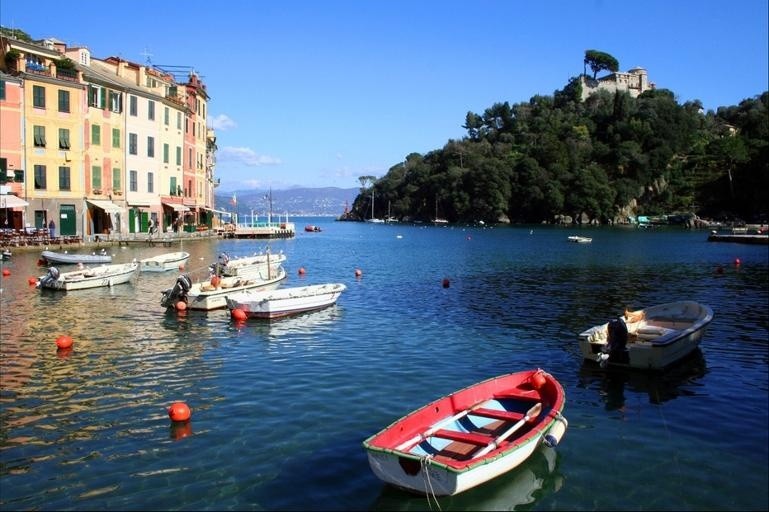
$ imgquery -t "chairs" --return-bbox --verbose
[0,228,82,248]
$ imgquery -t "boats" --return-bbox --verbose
[568,235,593,245]
[161,251,346,319]
[362,368,568,496]
[431,193,450,223]
[577,300,714,369]
[364,190,399,223]
[36,263,138,290]
[708,224,769,245]
[140,252,191,271]
[305,225,321,232]
[41,251,112,263]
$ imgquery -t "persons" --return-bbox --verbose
[147,216,184,235]
[49,218,55,239]
[223,221,235,233]
